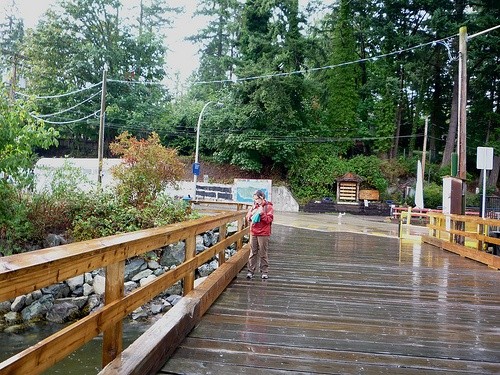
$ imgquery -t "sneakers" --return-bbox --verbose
[261,273,268,280]
[246,272,254,279]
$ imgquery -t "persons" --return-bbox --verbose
[245,190,275,280]
